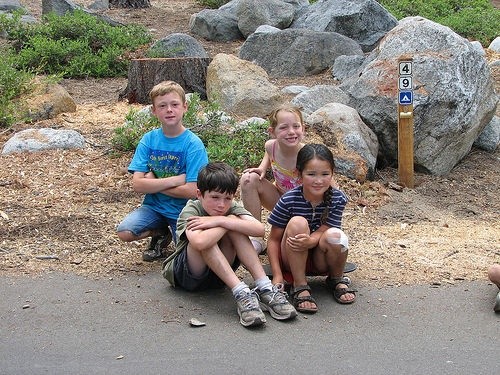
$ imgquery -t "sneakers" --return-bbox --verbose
[255,282,297,319]
[142,228,172,262]
[234,286,267,326]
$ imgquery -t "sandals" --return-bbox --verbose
[326,277,358,304]
[289,284,318,312]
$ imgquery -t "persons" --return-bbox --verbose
[239,105,337,258]
[266,144,358,313]
[162,163,299,327]
[488,263,500,314]
[115,80,210,262]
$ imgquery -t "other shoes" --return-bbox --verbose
[249,237,267,257]
